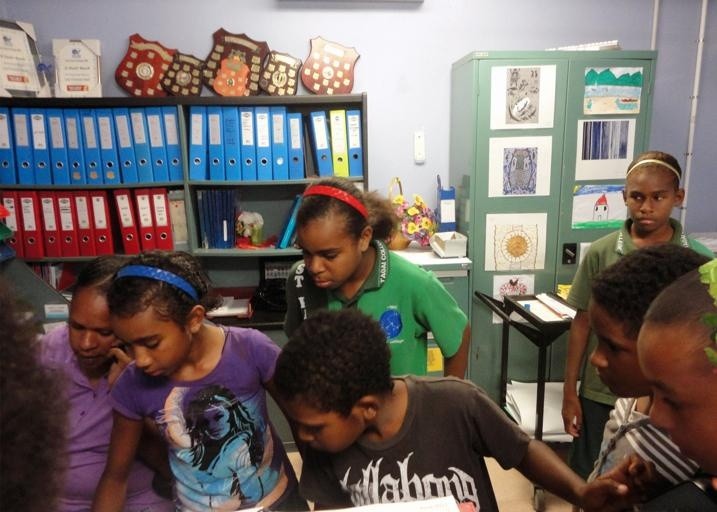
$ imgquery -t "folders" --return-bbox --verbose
[188,100,361,180]
[0,106,182,185]
[2,192,186,256]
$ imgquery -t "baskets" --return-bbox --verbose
[380,178,413,250]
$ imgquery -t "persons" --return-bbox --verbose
[636,256,716,512]
[554,148,712,477]
[24,255,178,512]
[268,305,659,511]
[279,175,467,382]
[85,245,314,512]
[582,241,710,487]
[167,385,264,512]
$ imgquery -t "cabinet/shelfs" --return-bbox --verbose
[451,48,659,408]
[389,247,473,380]
[0,92,369,453]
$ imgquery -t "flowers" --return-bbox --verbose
[392,194,436,247]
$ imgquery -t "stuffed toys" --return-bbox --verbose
[236,211,263,239]
